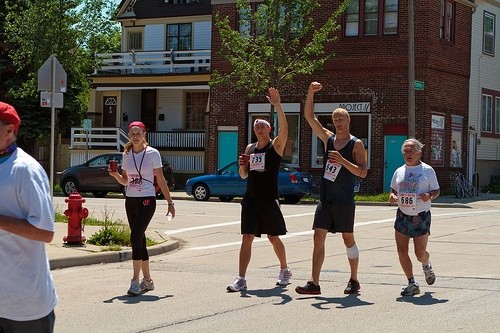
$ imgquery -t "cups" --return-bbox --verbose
[239,154,250,166]
[328,150,337,164]
[109,160,119,172]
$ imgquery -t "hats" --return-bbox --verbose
[129,121,145,133]
[0,102,21,133]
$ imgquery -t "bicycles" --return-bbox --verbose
[451,169,475,199]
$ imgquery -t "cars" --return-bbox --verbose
[60,153,174,200]
[185,159,314,205]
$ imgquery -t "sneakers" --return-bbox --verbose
[127,279,141,294]
[295,281,321,294]
[139,278,154,291]
[226,274,248,291]
[276,268,292,285]
[345,278,361,293]
[400,282,420,296]
[422,262,436,285]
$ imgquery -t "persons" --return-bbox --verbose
[295,81,367,294]
[390,139,441,295]
[226,86,293,291]
[0,102,58,333]
[108,121,176,295]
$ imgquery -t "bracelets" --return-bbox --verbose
[167,203,174,206]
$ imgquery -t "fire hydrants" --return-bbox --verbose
[63,188,89,245]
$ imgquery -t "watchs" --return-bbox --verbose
[428,192,434,200]
